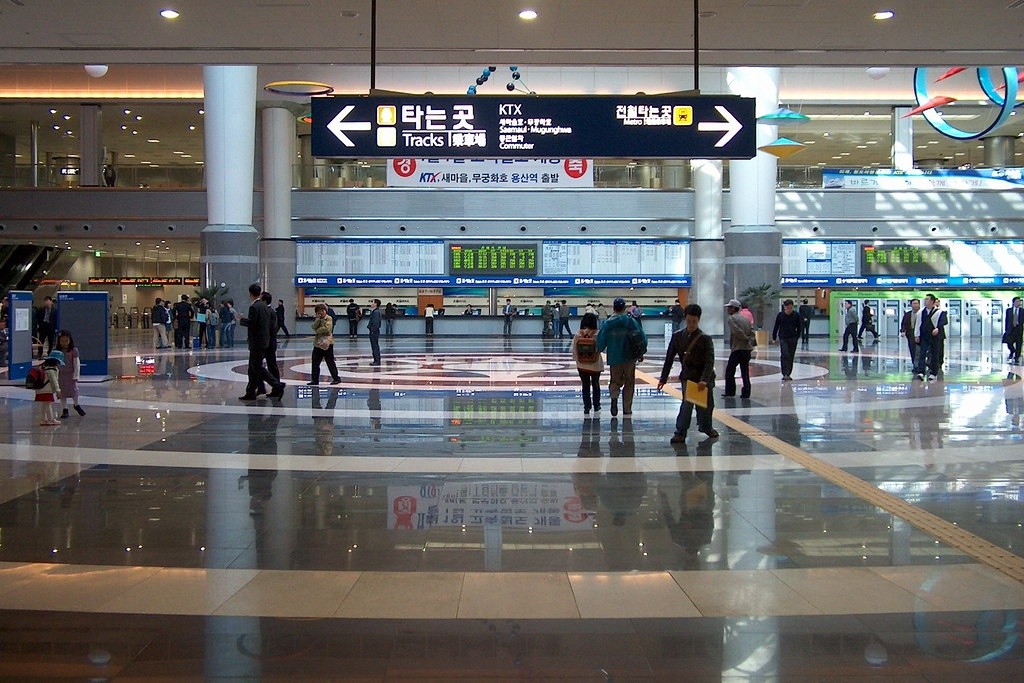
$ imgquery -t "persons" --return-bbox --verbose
[502,299,516,336]
[464,304,474,315]
[721,299,755,399]
[665,299,683,334]
[657,304,719,443]
[308,298,398,385]
[900,294,948,380]
[1005,297,1024,362]
[424,304,435,336]
[0,295,87,426]
[542,299,648,418]
[151,283,292,401]
[839,299,881,353]
[772,299,813,381]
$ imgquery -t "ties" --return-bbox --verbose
[1013,309,1018,327]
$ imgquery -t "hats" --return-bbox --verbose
[44,350,65,365]
[614,299,624,305]
[724,299,740,307]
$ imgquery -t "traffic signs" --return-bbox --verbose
[309,95,758,159]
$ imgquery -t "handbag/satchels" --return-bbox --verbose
[315,336,331,350]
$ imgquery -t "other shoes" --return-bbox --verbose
[61,408,69,418]
[782,375,792,381]
[40,418,61,426]
[838,348,847,351]
[851,347,859,352]
[239,383,285,400]
[74,404,86,416]
[698,428,719,437]
[917,373,924,381]
[330,378,341,385]
[929,374,937,380]
[610,399,632,417]
[584,402,600,415]
[739,394,750,398]
[370,363,380,366]
[1009,348,1019,360]
[156,344,233,349]
[721,391,735,396]
[307,381,319,385]
[670,436,685,443]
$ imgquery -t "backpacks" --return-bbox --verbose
[209,309,219,325]
[26,364,57,389]
[624,318,647,362]
[577,329,598,363]
